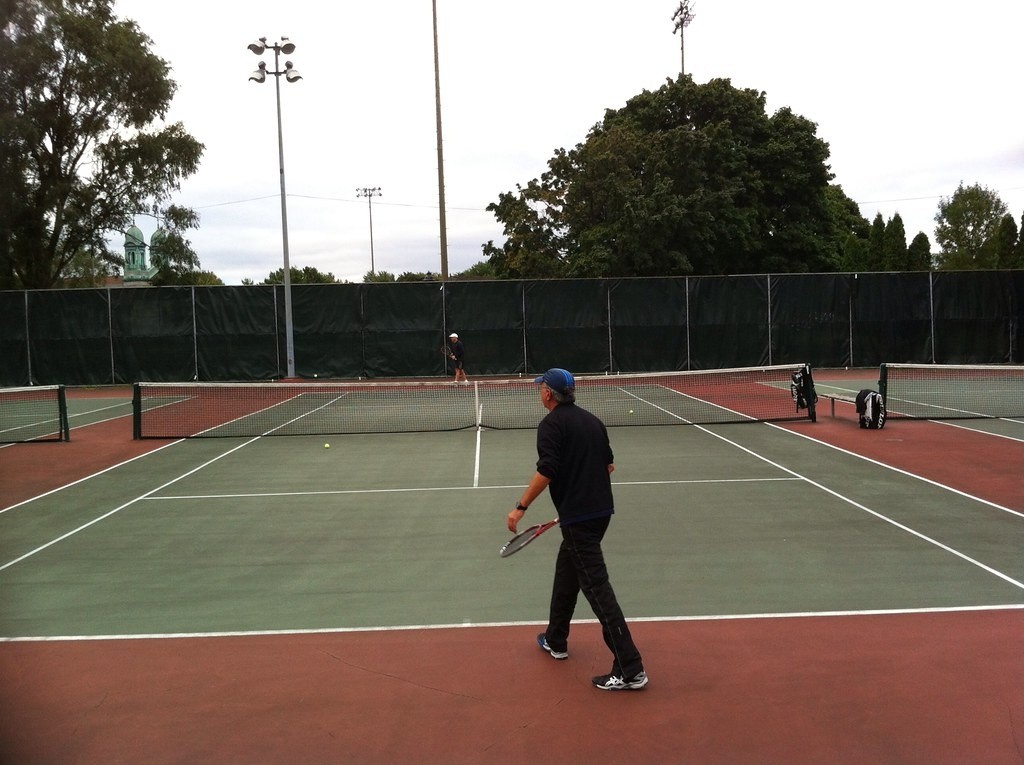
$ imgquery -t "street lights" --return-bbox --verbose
[356,187,382,276]
[245,37,304,378]
[671,0,697,72]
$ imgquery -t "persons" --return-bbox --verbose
[508,368,649,690]
[449,333,469,386]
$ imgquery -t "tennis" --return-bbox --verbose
[325,444,330,449]
[629,410,633,414]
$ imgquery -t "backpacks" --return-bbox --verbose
[791,366,818,409]
[859,392,886,429]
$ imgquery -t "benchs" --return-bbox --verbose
[821,393,856,418]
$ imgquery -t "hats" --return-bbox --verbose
[535,368,575,393]
[449,333,459,338]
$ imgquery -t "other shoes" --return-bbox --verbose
[451,380,459,383]
[461,379,468,383]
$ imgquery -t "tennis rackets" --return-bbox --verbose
[440,346,457,361]
[499,518,560,557]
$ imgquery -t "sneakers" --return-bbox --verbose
[537,633,568,659]
[592,670,648,689]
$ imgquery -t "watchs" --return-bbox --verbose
[515,501,527,511]
